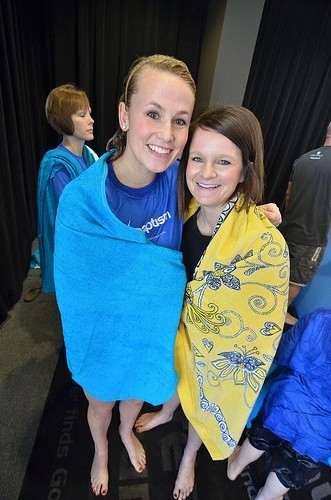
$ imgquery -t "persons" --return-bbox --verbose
[135,105,290,500]
[284,119,331,326]
[37,85,103,294]
[51,53,282,496]
[227,305,331,499]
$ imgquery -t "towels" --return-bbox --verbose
[36,149,86,295]
[183,192,288,462]
[53,149,188,406]
[247,308,331,468]
